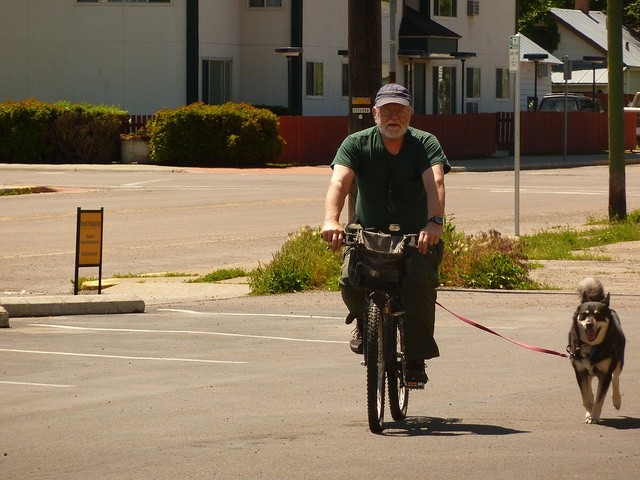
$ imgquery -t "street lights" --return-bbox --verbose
[273,47,304,116]
[583,55,606,113]
[396,49,426,97]
[450,51,477,115]
[524,53,548,112]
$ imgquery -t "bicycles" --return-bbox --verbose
[319,228,425,432]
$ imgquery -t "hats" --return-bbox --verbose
[374,82,412,108]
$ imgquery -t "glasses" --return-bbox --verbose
[379,106,409,118]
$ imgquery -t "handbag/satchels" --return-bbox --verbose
[348,230,408,294]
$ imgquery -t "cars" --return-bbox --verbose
[537,92,607,112]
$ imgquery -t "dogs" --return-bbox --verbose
[567,276,627,424]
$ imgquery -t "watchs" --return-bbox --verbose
[427,216,443,226]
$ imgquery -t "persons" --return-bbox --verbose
[320,84,451,383]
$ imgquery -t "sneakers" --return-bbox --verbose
[350,326,363,352]
[404,360,428,383]
